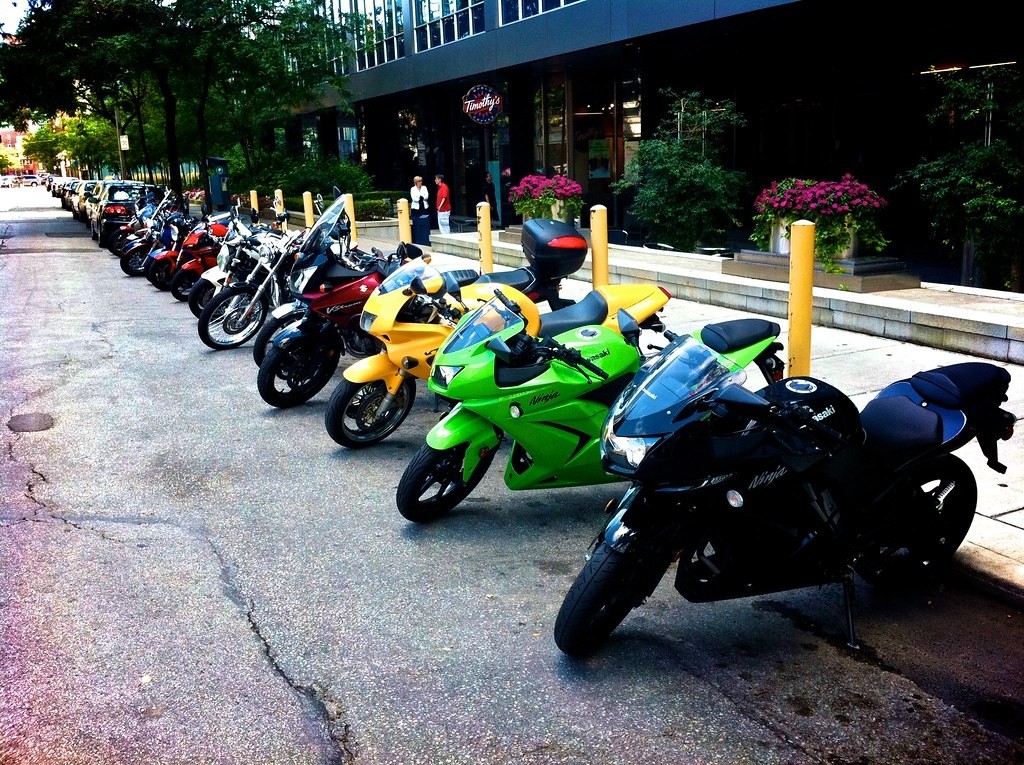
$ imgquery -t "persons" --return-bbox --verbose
[482,172,500,221]
[434,175,451,234]
[410,176,429,216]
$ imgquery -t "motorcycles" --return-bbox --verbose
[107,186,589,409]
[396,288,785,524]
[325,255,672,448]
[553,307,1017,656]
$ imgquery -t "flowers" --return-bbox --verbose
[508,174,586,220]
[749,172,891,273]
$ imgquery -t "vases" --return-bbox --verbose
[769,214,867,258]
[523,200,580,228]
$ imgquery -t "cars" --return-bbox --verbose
[85,180,165,248]
[0,173,144,228]
